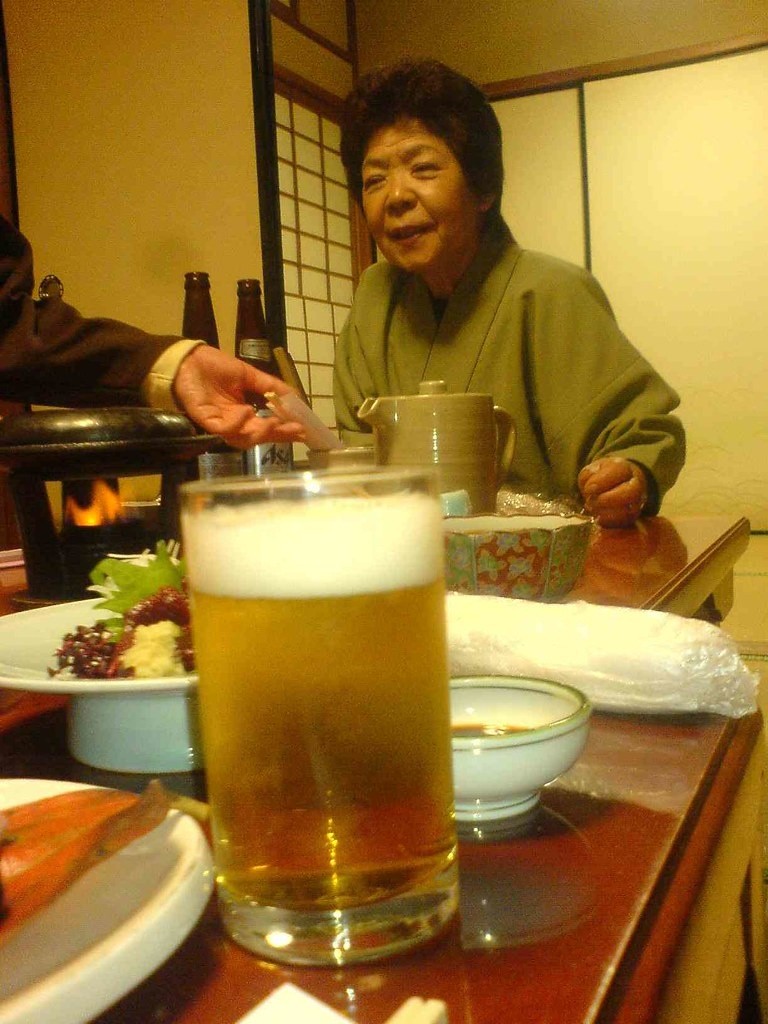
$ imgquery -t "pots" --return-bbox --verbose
[0,407,223,482]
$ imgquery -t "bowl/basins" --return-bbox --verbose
[450,671,590,822]
[444,512,594,603]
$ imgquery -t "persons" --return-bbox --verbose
[0,216,305,451]
[332,57,686,527]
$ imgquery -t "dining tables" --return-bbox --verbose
[1,515,767,1024]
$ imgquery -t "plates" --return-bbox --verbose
[1,598,199,775]
[0,776,214,1024]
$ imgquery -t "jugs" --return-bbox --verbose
[356,380,518,517]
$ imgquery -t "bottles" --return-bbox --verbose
[235,278,280,378]
[182,271,219,349]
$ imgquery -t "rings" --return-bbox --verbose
[627,503,632,511]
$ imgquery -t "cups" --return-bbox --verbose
[178,463,463,967]
